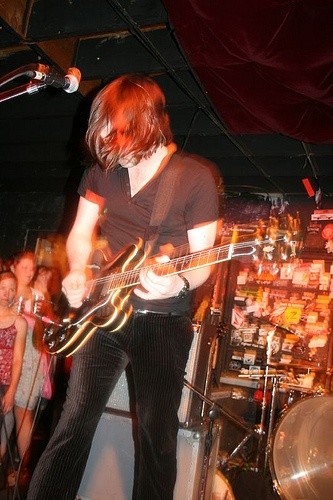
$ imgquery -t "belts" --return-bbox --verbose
[133,309,182,316]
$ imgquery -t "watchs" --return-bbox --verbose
[174,272,193,301]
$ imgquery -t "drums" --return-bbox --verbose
[265,391,333,500]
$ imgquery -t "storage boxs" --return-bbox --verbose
[76,323,222,500]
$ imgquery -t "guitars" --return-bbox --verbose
[41,235,293,359]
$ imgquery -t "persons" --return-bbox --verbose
[1,269,28,462]
[27,258,62,301]
[24,75,223,500]
[10,252,46,494]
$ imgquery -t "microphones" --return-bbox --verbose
[27,70,78,93]
[250,315,295,334]
